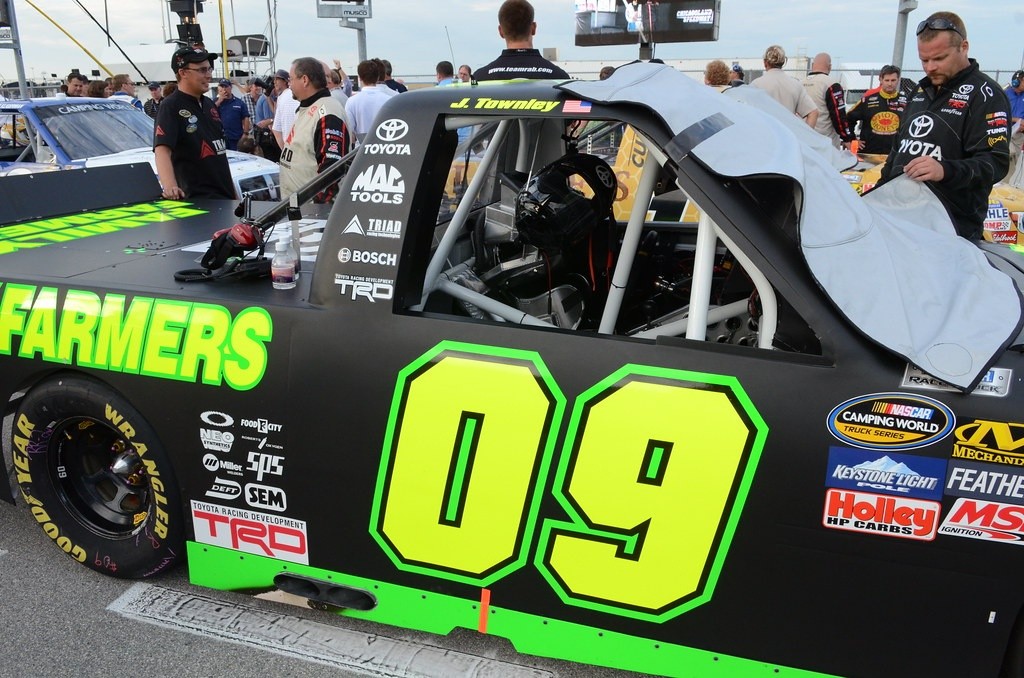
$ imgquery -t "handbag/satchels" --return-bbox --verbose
[257,128,279,147]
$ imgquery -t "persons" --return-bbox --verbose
[278,58,350,203]
[625,0,644,32]
[0,45,1024,204]
[152,46,239,200]
[472,0,572,180]
[875,11,1012,244]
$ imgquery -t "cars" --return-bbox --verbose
[0,77,1024,678]
[440,119,1024,245]
[1,95,282,204]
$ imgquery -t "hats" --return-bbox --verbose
[731,63,744,73]
[272,68,289,82]
[148,81,160,88]
[171,46,218,74]
[218,77,231,87]
[193,39,205,46]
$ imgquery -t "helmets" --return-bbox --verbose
[515,153,618,247]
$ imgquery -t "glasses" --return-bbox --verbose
[915,17,964,40]
[220,85,229,87]
[187,66,212,73]
